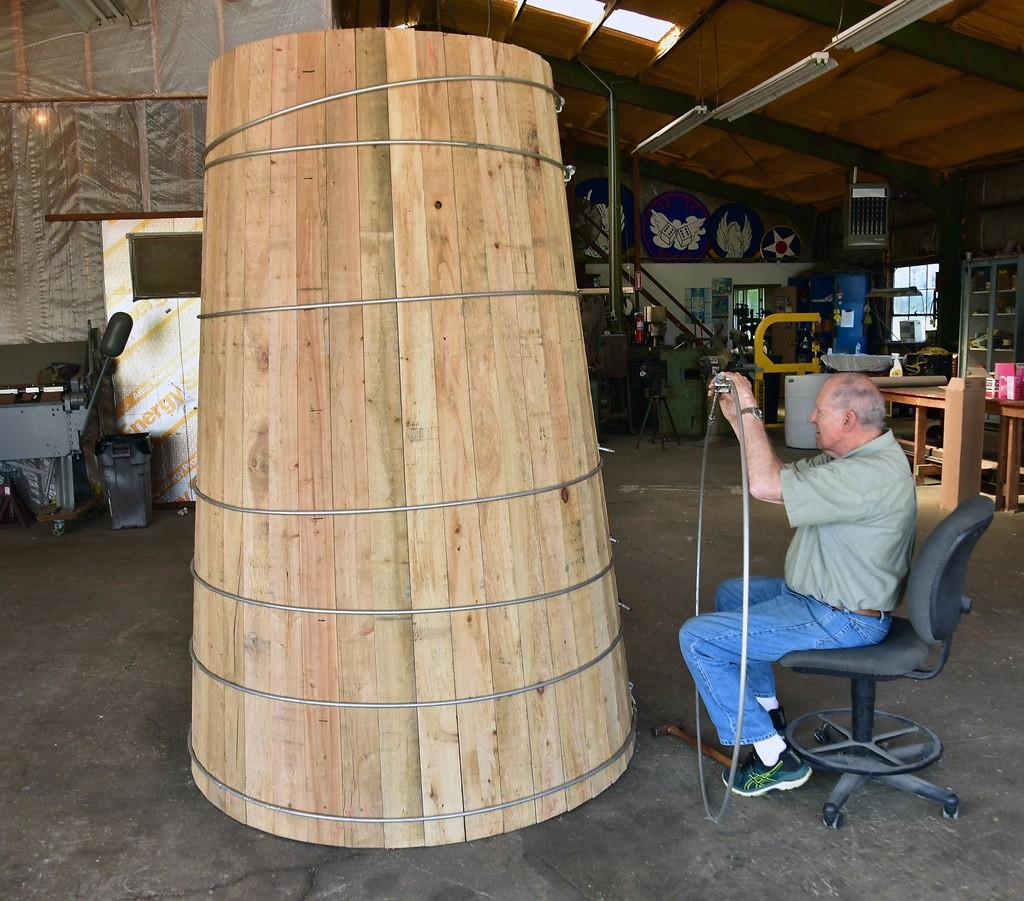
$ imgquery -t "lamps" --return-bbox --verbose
[823,0,954,54]
[627,104,714,157]
[845,166,857,185]
[863,286,923,298]
[864,299,874,324]
[712,50,839,124]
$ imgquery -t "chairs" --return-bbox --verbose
[776,493,996,829]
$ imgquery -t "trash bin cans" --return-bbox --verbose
[95,432,155,530]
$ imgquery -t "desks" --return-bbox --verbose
[878,383,1024,510]
[882,337,934,355]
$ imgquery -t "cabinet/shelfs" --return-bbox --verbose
[956,253,1024,378]
[790,267,869,375]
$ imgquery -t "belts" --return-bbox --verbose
[850,608,889,617]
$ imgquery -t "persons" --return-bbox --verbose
[579,280,605,334]
[679,371,917,796]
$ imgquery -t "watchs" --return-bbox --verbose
[741,407,764,420]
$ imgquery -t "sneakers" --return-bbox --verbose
[768,706,788,740]
[722,745,812,797]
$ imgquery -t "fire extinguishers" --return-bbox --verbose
[633,312,647,344]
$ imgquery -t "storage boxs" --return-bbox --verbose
[967,360,1024,402]
[939,376,986,511]
